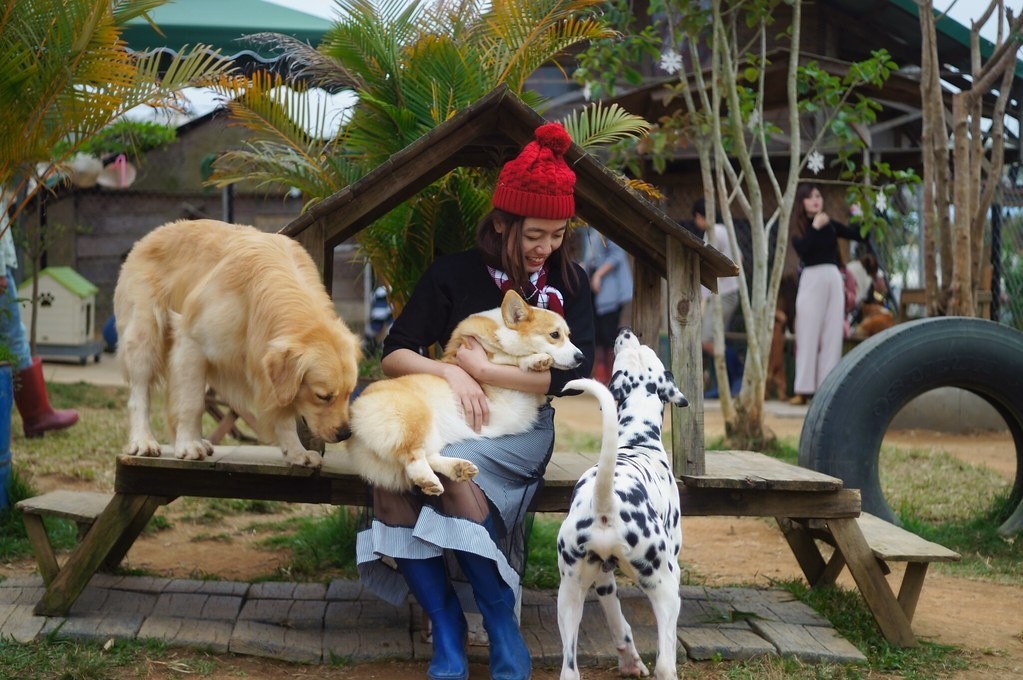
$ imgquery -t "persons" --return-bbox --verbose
[691,201,746,401]
[581,223,635,382]
[355,122,597,680]
[847,254,889,325]
[0,187,81,437]
[788,181,867,406]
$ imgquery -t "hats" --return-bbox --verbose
[492,123,576,221]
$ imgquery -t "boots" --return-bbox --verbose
[12,357,80,438]
[454,514,533,680]
[394,555,469,680]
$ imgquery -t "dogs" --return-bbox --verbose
[556,326,691,680]
[317,288,587,497]
[118,217,365,470]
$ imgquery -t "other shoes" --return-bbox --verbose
[790,395,807,405]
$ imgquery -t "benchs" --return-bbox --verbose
[13,441,963,657]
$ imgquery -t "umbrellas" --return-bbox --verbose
[32,1,385,93]
